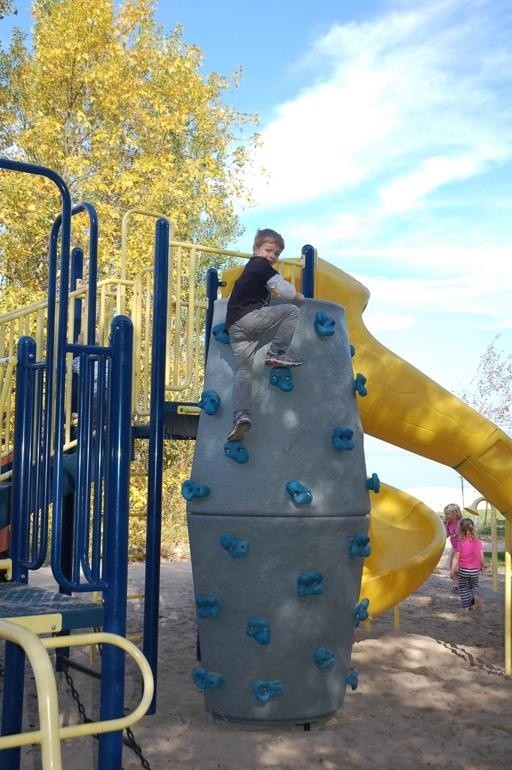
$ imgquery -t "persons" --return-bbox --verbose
[448,517,485,613]
[222,226,308,446]
[443,502,465,592]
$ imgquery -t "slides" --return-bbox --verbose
[219,258,512,530]
[356,474,448,630]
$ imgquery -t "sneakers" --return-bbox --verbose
[451,585,460,596]
[225,411,253,444]
[263,347,306,370]
